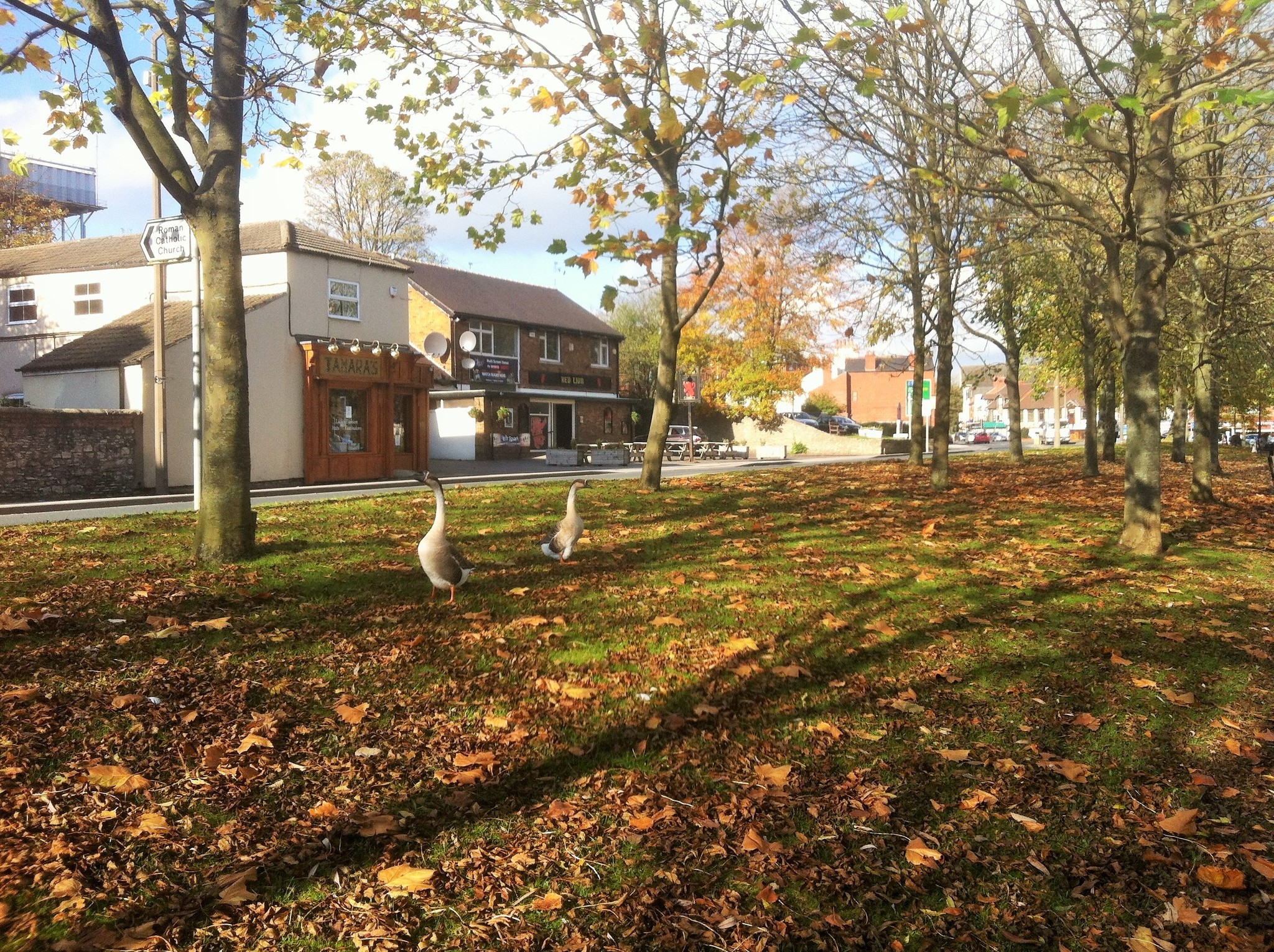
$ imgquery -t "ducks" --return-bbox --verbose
[541,479,593,565]
[409,470,477,603]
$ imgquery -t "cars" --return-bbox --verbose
[1255,432,1274,456]
[954,432,968,441]
[1160,419,1173,440]
[965,433,976,445]
[1244,434,1258,444]
[780,412,820,430]
[986,432,1007,442]
[816,416,862,434]
[633,425,710,456]
[974,432,990,444]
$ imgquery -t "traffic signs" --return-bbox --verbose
[141,215,195,263]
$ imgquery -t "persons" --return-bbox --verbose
[1114,419,1121,444]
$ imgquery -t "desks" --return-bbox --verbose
[576,442,735,461]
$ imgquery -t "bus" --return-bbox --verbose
[1035,418,1071,444]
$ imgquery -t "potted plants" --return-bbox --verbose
[718,438,749,459]
[591,438,630,467]
[755,438,788,460]
[496,407,510,420]
[469,407,482,418]
[631,412,639,423]
[545,437,583,466]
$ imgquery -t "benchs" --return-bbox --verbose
[582,449,737,457]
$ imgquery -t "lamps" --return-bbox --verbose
[382,343,399,359]
[363,340,381,356]
[318,337,338,354]
[342,338,360,355]
